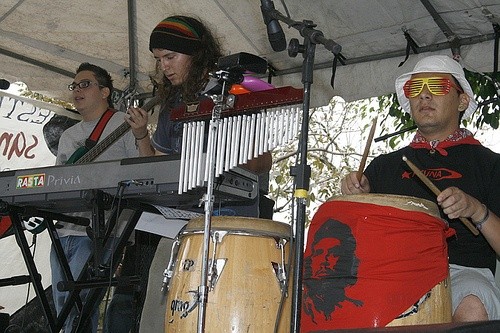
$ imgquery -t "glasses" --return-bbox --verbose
[401,76,464,98]
[68,81,104,91]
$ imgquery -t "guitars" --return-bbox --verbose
[0,92,168,241]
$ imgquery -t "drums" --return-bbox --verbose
[299,193,452,333]
[164,215,295,333]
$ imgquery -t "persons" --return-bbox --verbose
[340,54,500,324]
[49,61,155,333]
[138,15,272,333]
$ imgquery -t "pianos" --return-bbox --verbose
[0,153,259,333]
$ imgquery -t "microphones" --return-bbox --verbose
[261,0,286,52]
[0,79,10,89]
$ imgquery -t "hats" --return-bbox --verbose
[394,55,478,119]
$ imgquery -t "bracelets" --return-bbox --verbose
[136,130,148,140]
[472,203,490,225]
